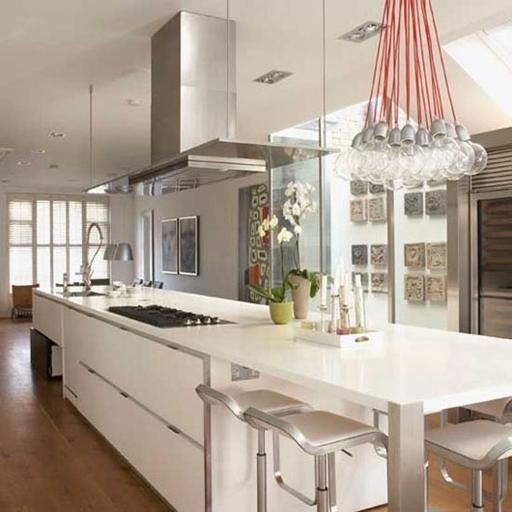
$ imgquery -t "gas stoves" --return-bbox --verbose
[106,304,239,328]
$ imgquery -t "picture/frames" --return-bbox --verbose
[160,218,178,275]
[179,214,199,276]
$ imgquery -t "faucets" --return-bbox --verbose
[63,273,68,292]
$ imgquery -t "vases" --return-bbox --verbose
[269,302,295,323]
[287,273,312,318]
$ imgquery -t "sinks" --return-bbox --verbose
[54,290,108,298]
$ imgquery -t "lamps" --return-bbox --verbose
[103,197,134,261]
[334,1,489,192]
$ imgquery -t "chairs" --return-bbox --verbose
[423,397,512,511]
[10,284,40,322]
[243,405,389,511]
[195,383,317,512]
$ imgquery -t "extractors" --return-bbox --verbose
[81,9,341,199]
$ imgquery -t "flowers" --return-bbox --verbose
[247,216,292,302]
[282,180,320,298]
